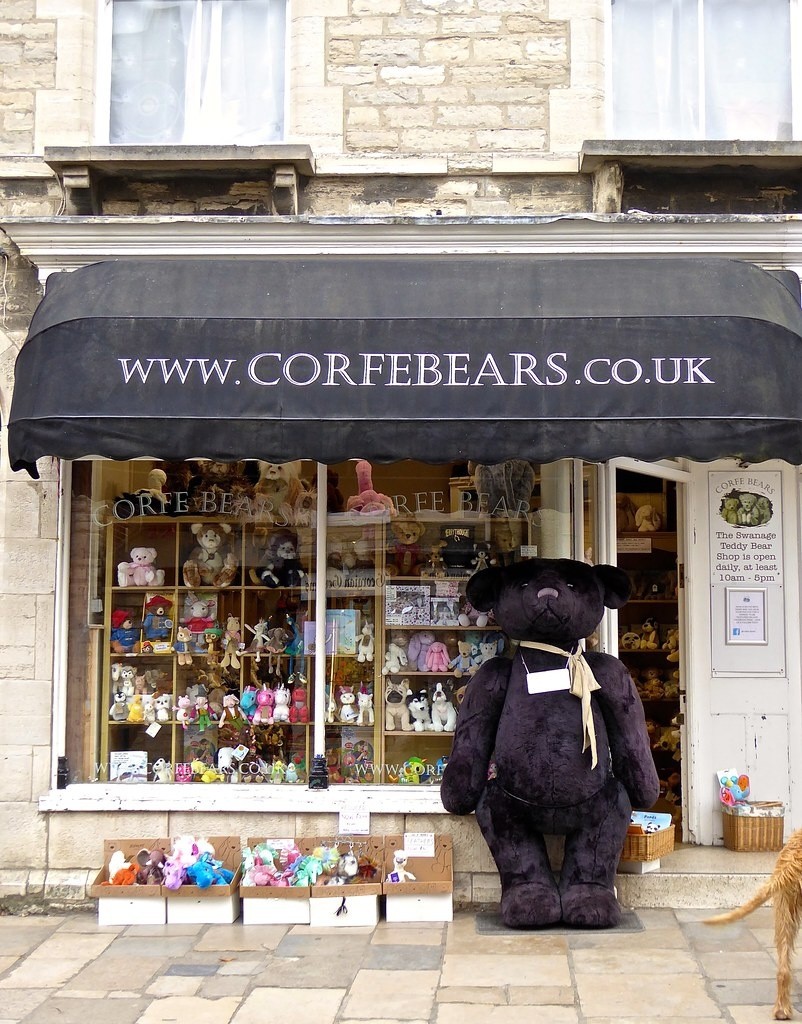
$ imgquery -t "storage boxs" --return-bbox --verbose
[383,833,455,924]
[239,837,310,925]
[308,835,385,929]
[162,837,243,925]
[91,838,173,926]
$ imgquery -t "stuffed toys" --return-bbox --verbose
[96,456,680,892]
[439,557,658,929]
[721,491,772,529]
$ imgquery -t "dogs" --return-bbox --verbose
[700,829,802,1020]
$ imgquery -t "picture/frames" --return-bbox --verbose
[726,587,769,646]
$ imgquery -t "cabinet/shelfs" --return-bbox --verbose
[380,507,533,785]
[100,511,383,785]
[617,530,681,842]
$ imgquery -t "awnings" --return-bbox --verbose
[8,253,802,481]
